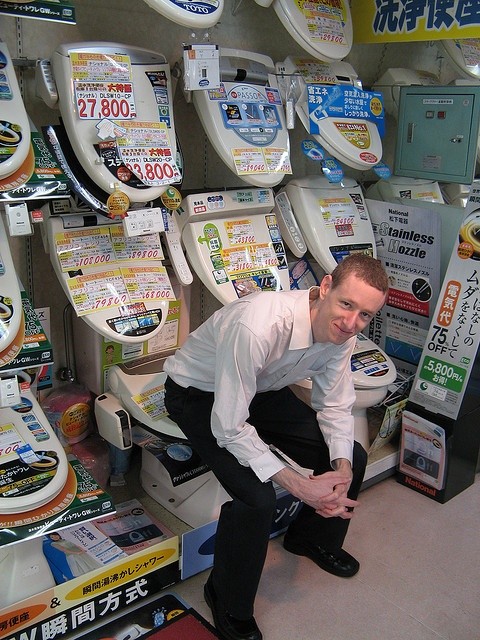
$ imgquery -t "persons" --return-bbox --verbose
[163,253,389,640]
[48,532,104,577]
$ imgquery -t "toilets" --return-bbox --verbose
[63,306,233,530]
[286,259,397,454]
[0,376,69,609]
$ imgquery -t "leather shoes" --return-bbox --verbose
[204,581,263,640]
[284,530,360,578]
